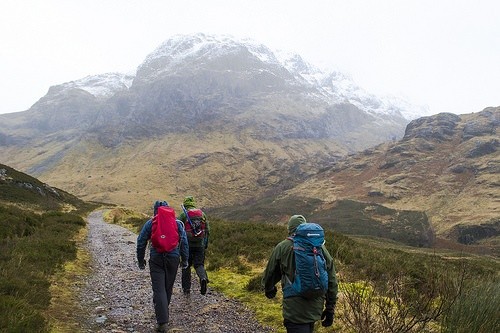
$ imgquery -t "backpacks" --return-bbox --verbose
[185,209,205,239]
[152,206,179,253]
[284,223,328,296]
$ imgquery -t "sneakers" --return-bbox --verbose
[200,279,207,295]
[158,324,168,332]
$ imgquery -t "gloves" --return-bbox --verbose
[321,311,334,327]
[138,259,147,269]
[265,286,278,299]
[179,261,188,269]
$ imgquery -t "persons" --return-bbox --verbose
[263,215,338,333]
[177,195,210,296]
[137,201,189,330]
